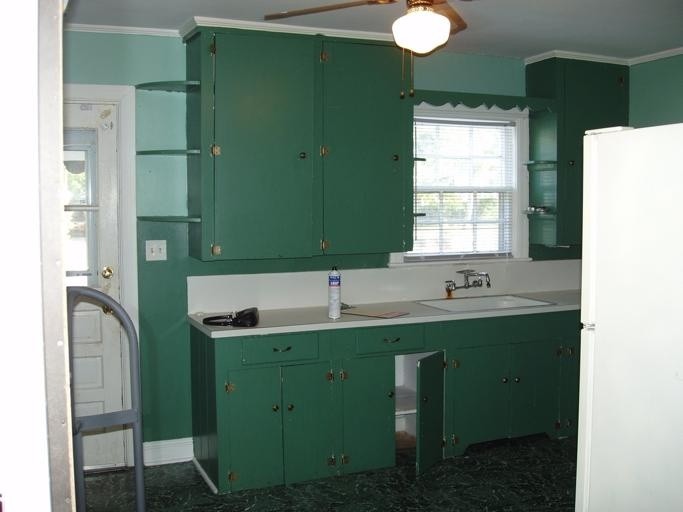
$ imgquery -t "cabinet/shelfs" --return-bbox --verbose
[191,307,581,495]
[136,28,628,262]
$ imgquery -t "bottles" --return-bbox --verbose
[327,266,342,319]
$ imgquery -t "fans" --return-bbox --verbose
[263,0,467,40]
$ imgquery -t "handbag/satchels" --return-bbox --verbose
[203,306,259,327]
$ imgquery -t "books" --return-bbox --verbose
[342,306,410,319]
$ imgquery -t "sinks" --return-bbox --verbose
[414,294,557,313]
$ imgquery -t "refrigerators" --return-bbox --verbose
[574,122,683,512]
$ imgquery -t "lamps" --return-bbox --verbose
[390,4,450,54]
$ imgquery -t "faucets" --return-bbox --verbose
[464,270,492,290]
